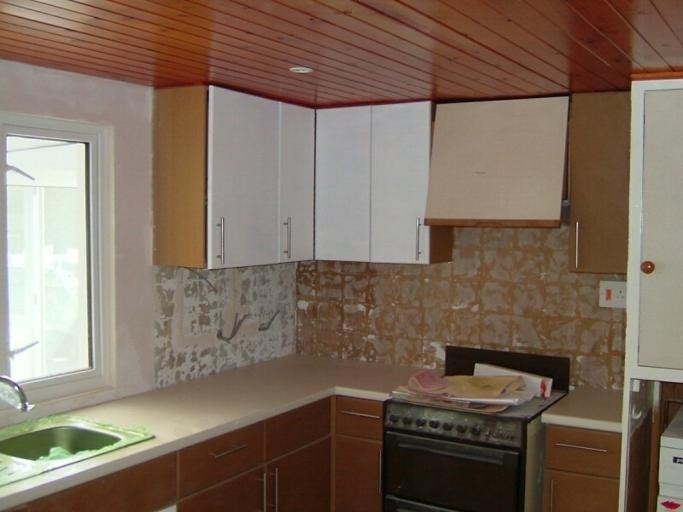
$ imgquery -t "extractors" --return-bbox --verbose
[424,96,569,236]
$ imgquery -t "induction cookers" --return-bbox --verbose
[384,376,566,449]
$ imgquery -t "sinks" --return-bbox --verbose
[0,415,154,473]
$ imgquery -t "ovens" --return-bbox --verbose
[382,431,524,511]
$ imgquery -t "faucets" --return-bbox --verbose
[0,376,34,413]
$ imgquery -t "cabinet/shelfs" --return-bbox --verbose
[567,90,631,275]
[0,395,382,512]
[541,423,621,512]
[152,85,314,271]
[624,77,682,385]
[314,100,451,265]
